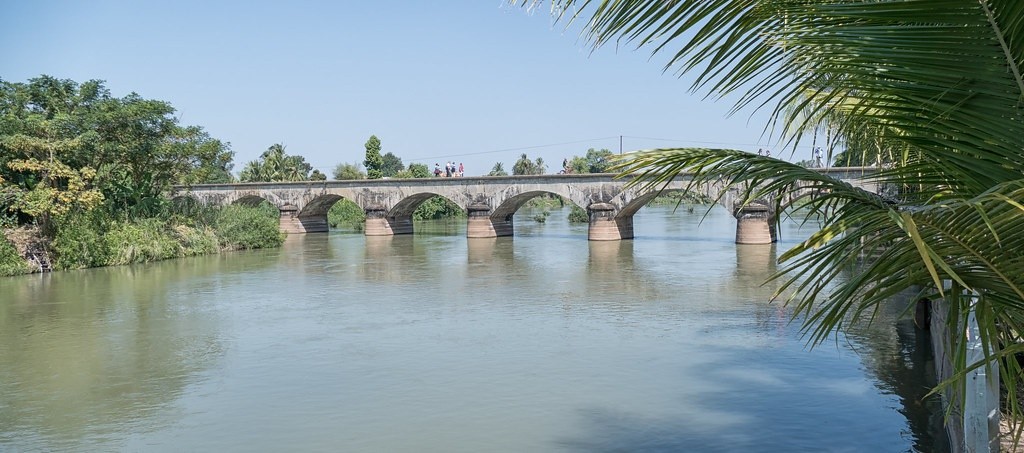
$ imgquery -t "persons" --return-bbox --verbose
[459,163,464,177]
[766,150,771,156]
[757,149,763,155]
[815,146,824,167]
[434,163,443,176]
[563,158,569,173]
[446,161,455,177]
